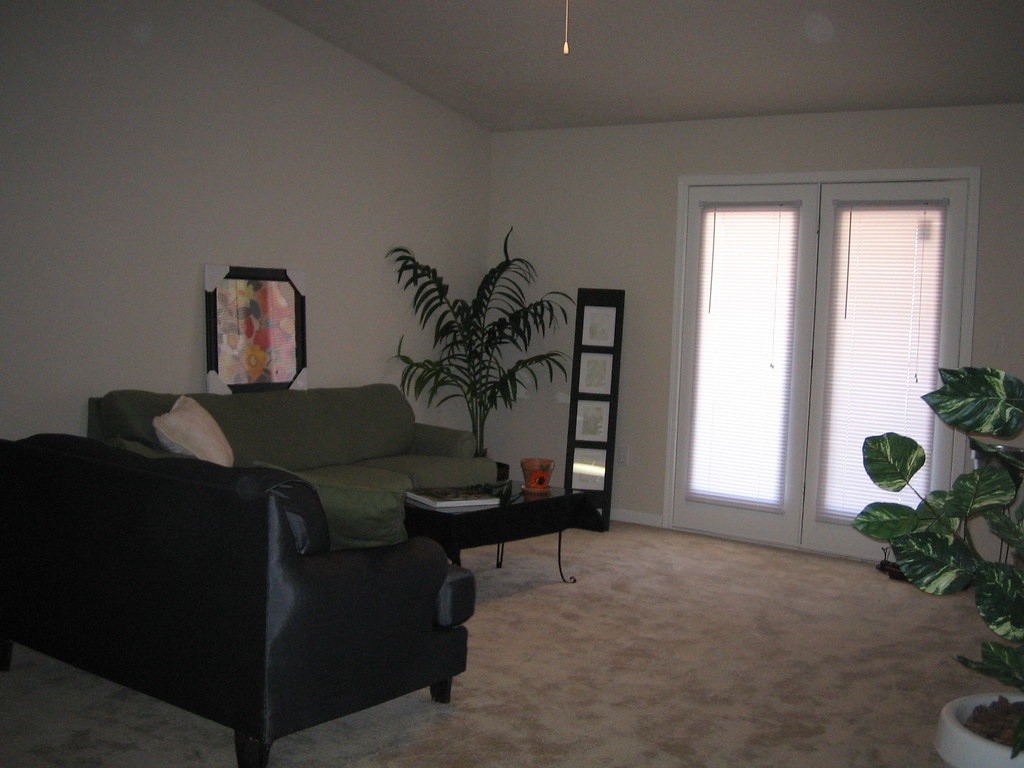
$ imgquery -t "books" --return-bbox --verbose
[406,486,501,507]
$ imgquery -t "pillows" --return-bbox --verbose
[240,458,408,548]
[152,395,235,468]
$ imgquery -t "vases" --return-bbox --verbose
[521,458,555,494]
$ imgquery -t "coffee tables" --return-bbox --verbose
[404,481,585,584]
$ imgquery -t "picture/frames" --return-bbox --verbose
[204,263,308,394]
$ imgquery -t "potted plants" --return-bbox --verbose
[850,367,1024,768]
[384,225,576,481]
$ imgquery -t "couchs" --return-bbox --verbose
[0,432,477,768]
[86,384,498,490]
